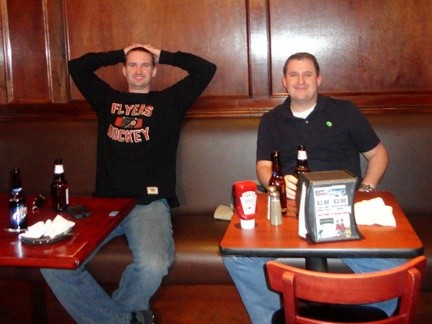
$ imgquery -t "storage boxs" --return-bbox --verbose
[298,168,363,243]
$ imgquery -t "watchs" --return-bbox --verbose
[358,184,376,193]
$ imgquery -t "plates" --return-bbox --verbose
[18,225,73,245]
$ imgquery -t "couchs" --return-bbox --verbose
[0,112,432,292]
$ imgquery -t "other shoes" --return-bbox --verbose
[130,310,155,324]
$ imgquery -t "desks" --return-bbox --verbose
[220,190,425,305]
[0,193,137,324]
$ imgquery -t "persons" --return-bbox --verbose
[221,51,410,324]
[38,43,218,324]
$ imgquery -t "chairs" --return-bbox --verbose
[264,255,428,324]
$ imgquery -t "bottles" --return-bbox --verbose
[9,168,27,230]
[233,181,257,229]
[296,142,310,217]
[51,158,70,212]
[264,185,277,222]
[270,192,283,226]
[268,150,288,215]
